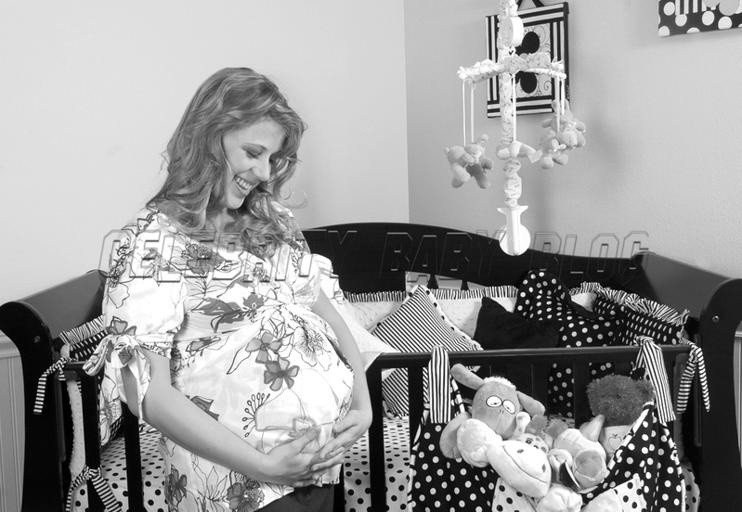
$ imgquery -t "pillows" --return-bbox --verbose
[473,296,560,421]
[363,288,485,416]
[512,269,624,416]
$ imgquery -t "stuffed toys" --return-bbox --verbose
[439,363,654,512]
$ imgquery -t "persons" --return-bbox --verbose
[82,65,375,512]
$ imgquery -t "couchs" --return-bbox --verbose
[0,221,741,511]
